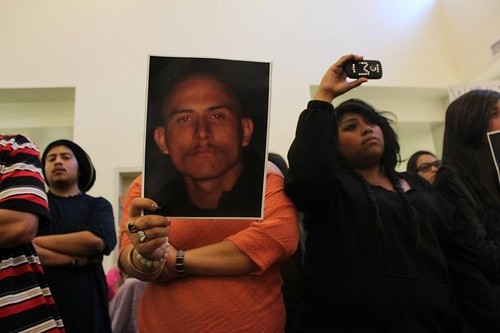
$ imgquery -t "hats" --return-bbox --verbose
[41,139,96,193]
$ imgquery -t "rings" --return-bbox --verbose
[137,231,147,242]
[126,220,138,232]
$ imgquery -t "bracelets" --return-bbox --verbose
[126,248,167,279]
[132,249,164,268]
[175,248,187,278]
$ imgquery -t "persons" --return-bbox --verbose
[283,54,465,333]
[144,71,264,217]
[30,139,117,333]
[431,89,500,333]
[116,160,299,333]
[0,133,66,333]
[405,150,440,185]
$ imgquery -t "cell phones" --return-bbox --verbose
[345,60,382,79]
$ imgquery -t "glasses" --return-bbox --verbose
[416,160,443,173]
